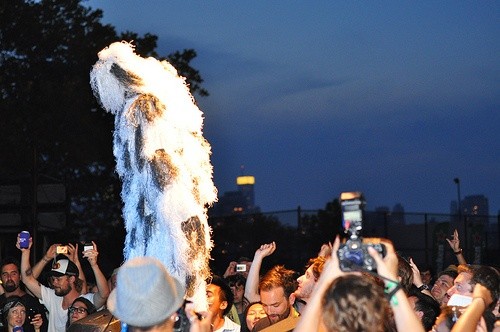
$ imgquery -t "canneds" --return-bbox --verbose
[13,325,23,332]
[22,231,29,233]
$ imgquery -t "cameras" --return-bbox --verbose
[337,191,385,272]
[235,264,247,271]
[56,246,68,253]
[84,246,93,251]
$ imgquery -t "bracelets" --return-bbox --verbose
[387,283,402,300]
[454,251,463,255]
[418,283,428,291]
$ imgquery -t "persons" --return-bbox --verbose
[0,229,500,332]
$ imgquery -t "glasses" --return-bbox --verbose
[68,307,89,314]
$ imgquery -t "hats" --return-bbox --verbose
[107,256,186,327]
[43,259,79,276]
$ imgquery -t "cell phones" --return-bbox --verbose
[19,232,30,249]
